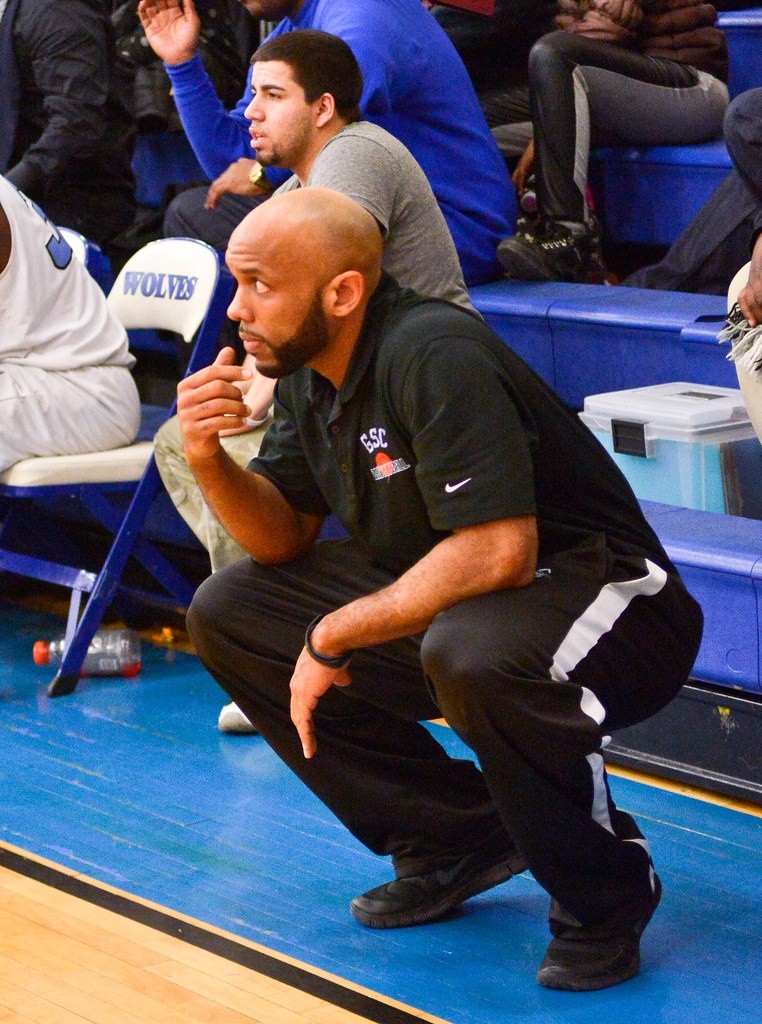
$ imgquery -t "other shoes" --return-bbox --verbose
[351,832,527,926]
[538,872,662,988]
[218,701,258,733]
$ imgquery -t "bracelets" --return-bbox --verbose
[305,614,351,668]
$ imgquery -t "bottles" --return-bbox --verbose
[33,629,141,676]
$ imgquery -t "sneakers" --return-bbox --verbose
[496,214,603,284]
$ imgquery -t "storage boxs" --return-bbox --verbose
[577,381,762,520]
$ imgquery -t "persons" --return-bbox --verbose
[0,1,762,735]
[177,186,703,990]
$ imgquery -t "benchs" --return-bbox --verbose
[464,6,762,806]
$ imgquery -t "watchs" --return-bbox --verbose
[250,163,274,192]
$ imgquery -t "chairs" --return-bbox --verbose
[0,238,236,696]
